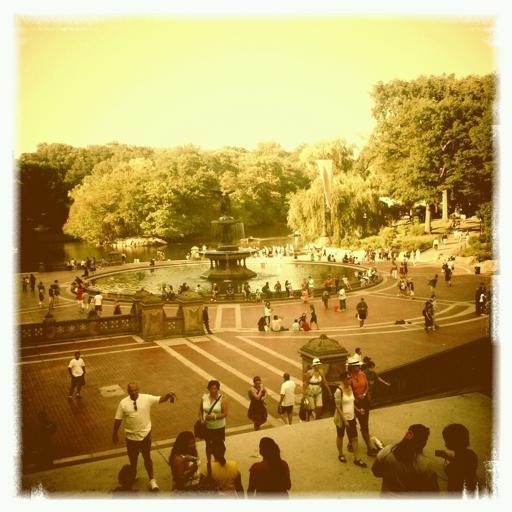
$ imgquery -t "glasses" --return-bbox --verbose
[134,401,137,411]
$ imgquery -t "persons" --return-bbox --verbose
[23,237,492,499]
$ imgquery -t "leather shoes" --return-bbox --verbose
[354,460,366,467]
[338,455,346,463]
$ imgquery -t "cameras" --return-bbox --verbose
[434,450,446,456]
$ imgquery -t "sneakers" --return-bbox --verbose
[348,443,353,452]
[149,479,159,490]
[368,448,377,456]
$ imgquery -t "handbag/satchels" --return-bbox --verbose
[194,420,208,440]
[299,400,307,421]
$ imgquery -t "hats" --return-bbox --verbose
[345,359,364,366]
[312,359,322,366]
